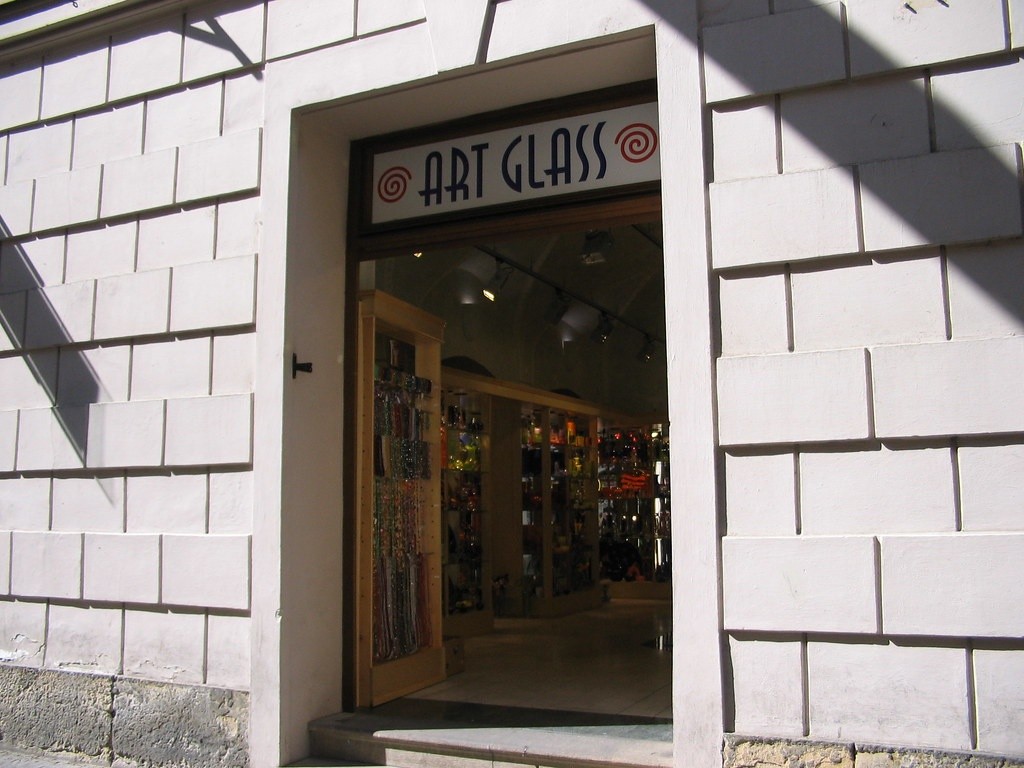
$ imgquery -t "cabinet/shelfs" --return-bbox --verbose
[340,288,670,710]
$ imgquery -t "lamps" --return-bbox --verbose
[591,312,615,345]
[635,335,656,363]
[544,291,571,325]
[482,261,517,301]
